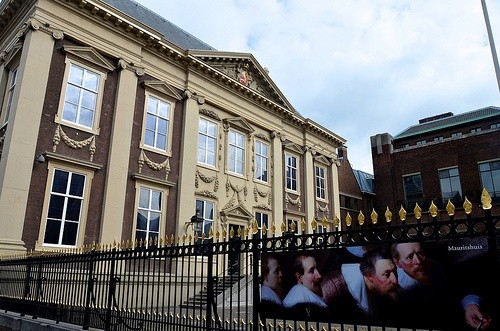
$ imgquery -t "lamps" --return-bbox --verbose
[184,205,204,234]
